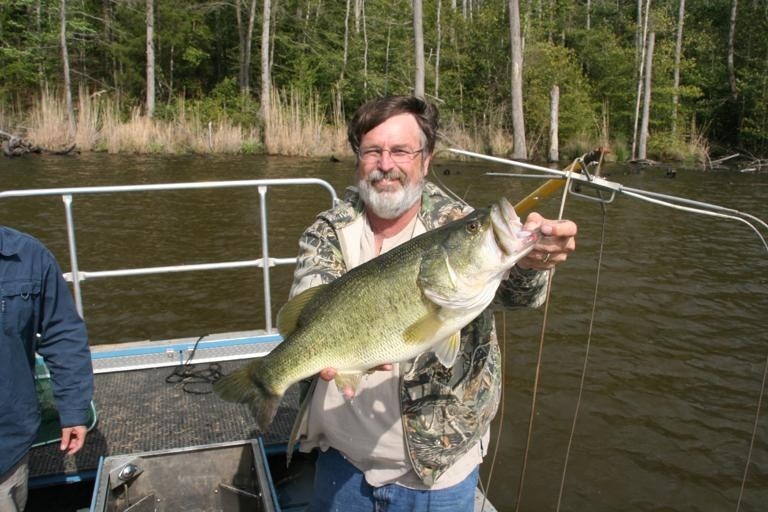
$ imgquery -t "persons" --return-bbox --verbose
[0,224,95,512]
[284,91,580,511]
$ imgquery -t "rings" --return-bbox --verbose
[539,250,552,265]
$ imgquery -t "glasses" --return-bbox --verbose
[355,145,429,164]
